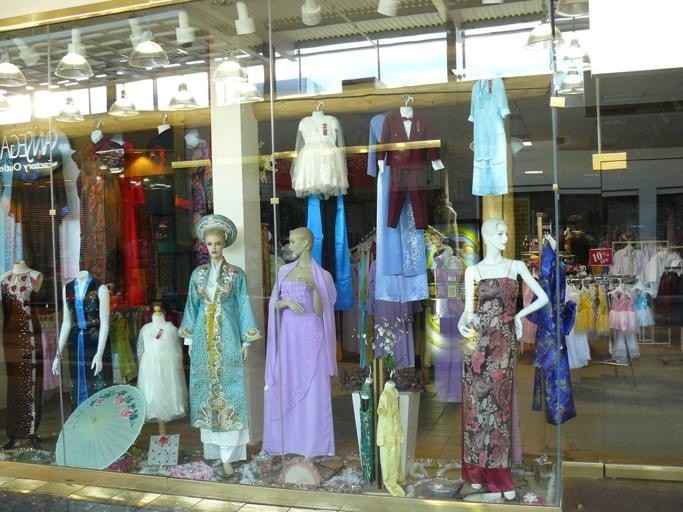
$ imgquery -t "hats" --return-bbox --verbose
[196,214,238,247]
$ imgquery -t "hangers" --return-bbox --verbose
[566,241,683,300]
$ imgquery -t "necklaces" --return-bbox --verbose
[480,256,504,267]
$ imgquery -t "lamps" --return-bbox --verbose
[556,1,589,20]
[556,70,586,96]
[0,0,265,124]
[299,1,324,27]
[524,21,566,48]
[377,2,400,18]
[562,40,589,67]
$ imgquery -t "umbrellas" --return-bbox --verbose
[53,383,146,471]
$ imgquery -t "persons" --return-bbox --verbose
[50,270,110,418]
[144,123,191,308]
[0,261,44,451]
[377,108,445,229]
[290,113,347,198]
[176,131,214,269]
[456,217,551,501]
[260,226,338,465]
[468,70,515,197]
[68,130,124,297]
[427,232,474,301]
[134,305,188,424]
[177,214,264,475]
